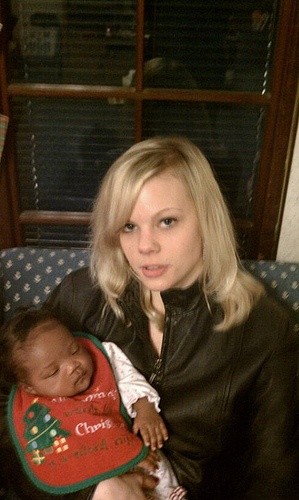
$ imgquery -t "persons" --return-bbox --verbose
[2,135,299,499]
[1,304,188,500]
